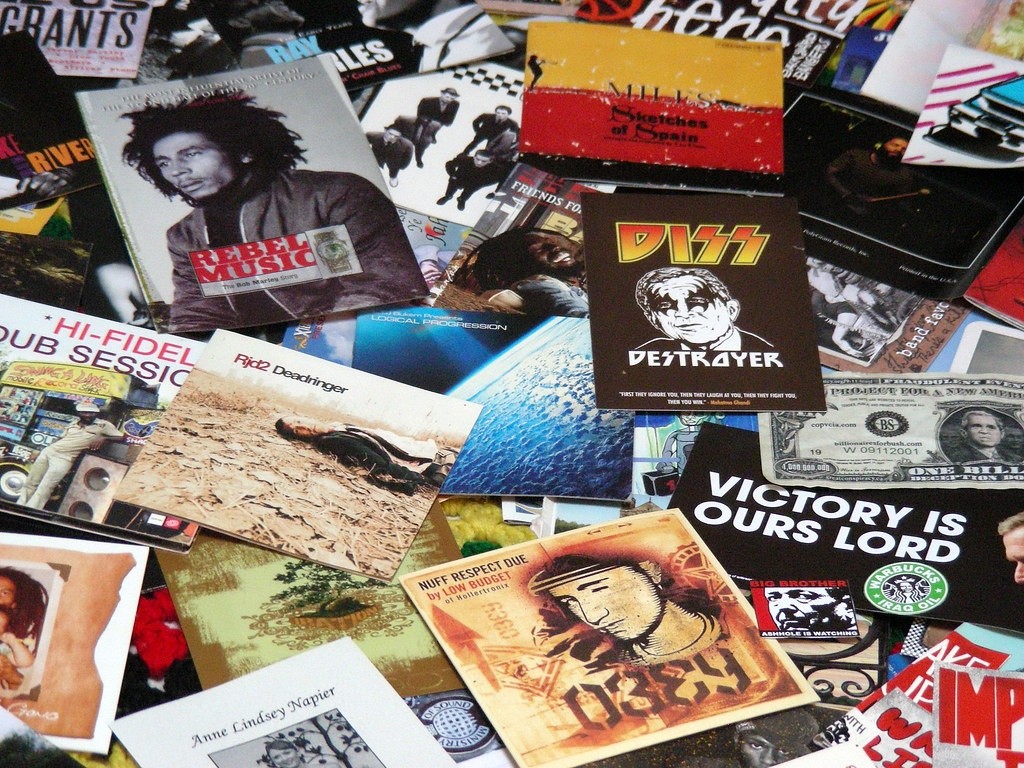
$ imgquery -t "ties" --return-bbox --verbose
[497,119,501,125]
[345,427,433,463]
[385,141,392,148]
[415,124,423,141]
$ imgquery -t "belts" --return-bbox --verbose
[426,451,442,478]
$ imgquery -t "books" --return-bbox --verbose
[0,0,1024,768]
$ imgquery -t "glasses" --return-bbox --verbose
[234,0,268,18]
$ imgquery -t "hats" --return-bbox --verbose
[76,402,100,412]
[441,87,460,97]
[384,124,406,137]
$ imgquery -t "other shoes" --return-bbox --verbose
[432,138,436,144]
[389,176,398,187]
[457,196,465,211]
[417,159,423,168]
[485,193,494,199]
[436,195,453,205]
[457,152,468,157]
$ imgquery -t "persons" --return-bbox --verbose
[16,402,125,510]
[461,227,590,318]
[683,710,818,768]
[417,88,460,144]
[811,289,870,363]
[356,0,514,73]
[266,740,341,768]
[0,94,81,210]
[806,258,899,331]
[0,566,49,691]
[365,124,413,187]
[116,89,430,332]
[395,115,441,169]
[201,0,326,71]
[455,105,520,156]
[825,137,911,231]
[0,606,33,668]
[275,416,461,489]
[998,511,1024,584]
[437,149,504,211]
